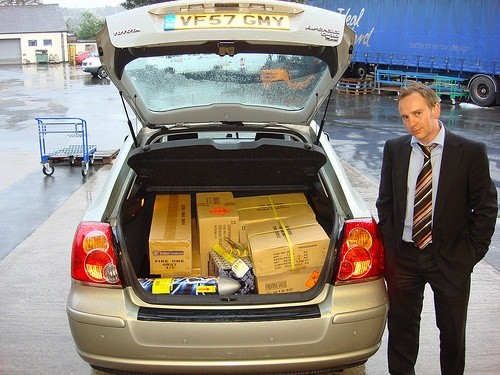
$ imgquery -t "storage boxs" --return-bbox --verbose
[149,192,330,295]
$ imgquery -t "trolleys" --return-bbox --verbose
[35,117,97,177]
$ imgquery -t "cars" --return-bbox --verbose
[76,49,95,64]
[65,0,389,375]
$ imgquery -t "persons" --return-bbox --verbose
[375,83,498,375]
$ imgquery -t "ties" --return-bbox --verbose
[411,142,439,250]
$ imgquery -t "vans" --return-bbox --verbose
[81,49,108,79]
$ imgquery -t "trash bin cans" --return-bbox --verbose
[35,50,48,63]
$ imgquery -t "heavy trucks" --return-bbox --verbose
[306,1,500,108]
